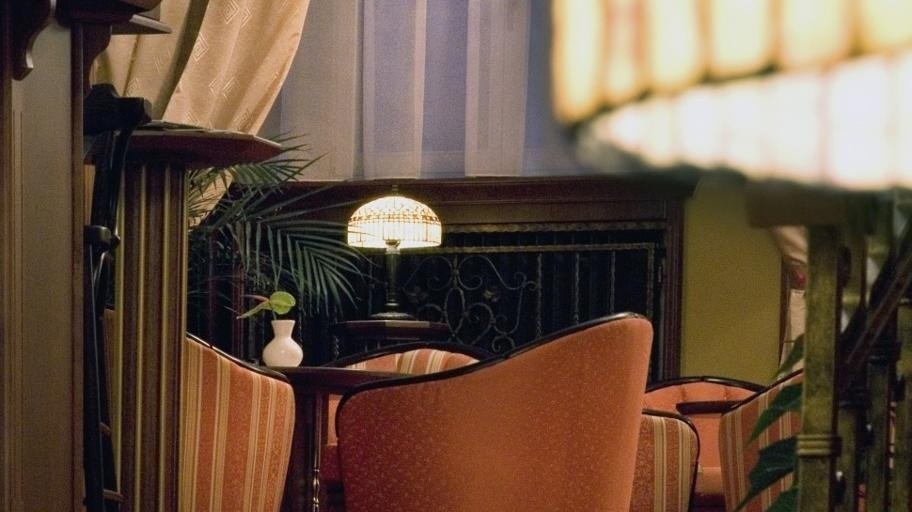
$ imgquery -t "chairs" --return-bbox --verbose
[332,312,653,511]
[99,306,295,511]
[313,342,500,512]
[629,409,700,512]
[719,366,893,512]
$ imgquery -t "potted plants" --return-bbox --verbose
[236,291,305,368]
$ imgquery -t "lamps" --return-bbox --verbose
[535,1,912,202]
[348,184,442,319]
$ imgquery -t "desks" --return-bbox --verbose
[676,400,745,418]
[642,375,770,506]
[273,367,417,511]
[341,318,448,351]
